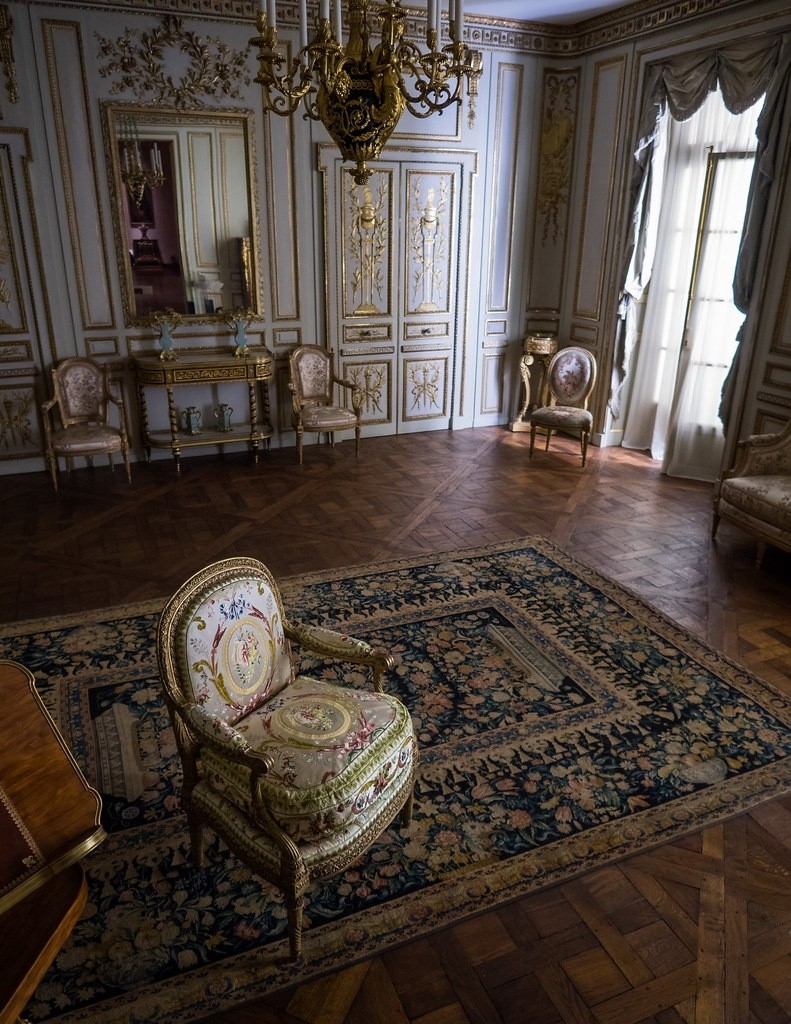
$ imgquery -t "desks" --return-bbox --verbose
[0,658,106,1024]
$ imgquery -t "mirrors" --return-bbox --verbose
[98,95,266,331]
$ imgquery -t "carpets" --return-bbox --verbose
[1,531,790,1024]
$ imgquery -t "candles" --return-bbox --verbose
[250,0,469,185]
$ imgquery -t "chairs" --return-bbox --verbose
[156,557,420,964]
[526,347,597,468]
[39,356,131,492]
[712,419,790,570]
[285,343,360,465]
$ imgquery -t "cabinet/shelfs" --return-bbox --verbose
[134,345,275,472]
